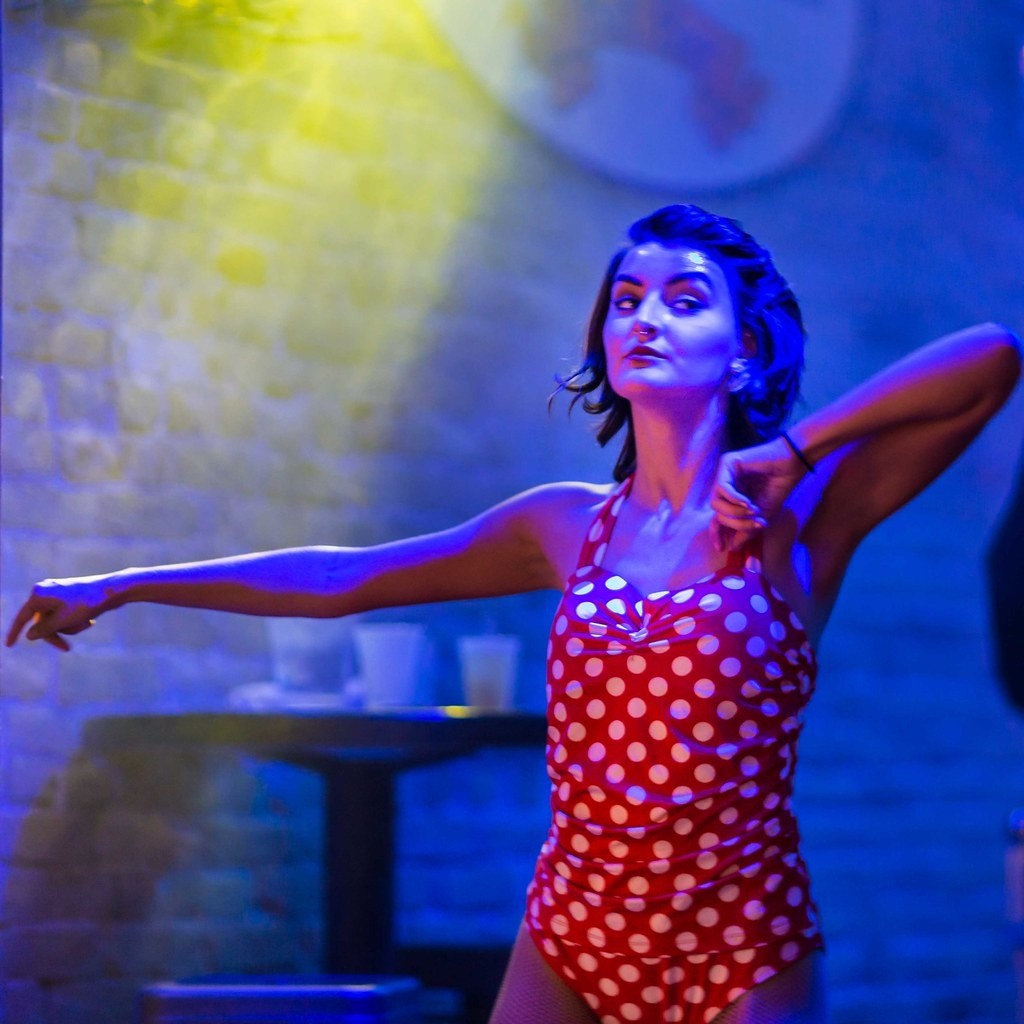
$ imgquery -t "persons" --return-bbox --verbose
[6,202,1023,1024]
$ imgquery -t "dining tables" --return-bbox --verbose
[86,696,544,1024]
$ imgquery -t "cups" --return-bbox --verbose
[351,618,432,710]
[267,616,353,689]
[458,633,518,707]
